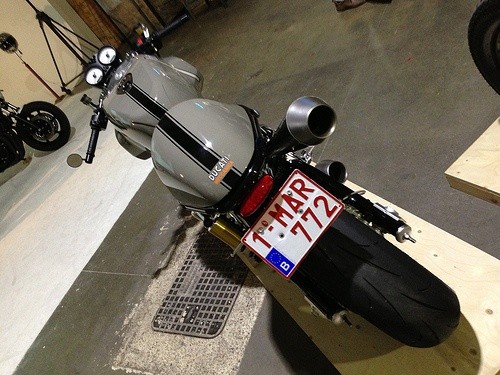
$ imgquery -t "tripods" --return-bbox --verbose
[25,0,100,96]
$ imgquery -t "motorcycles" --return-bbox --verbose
[0,90,70,172]
[67,14,460,347]
